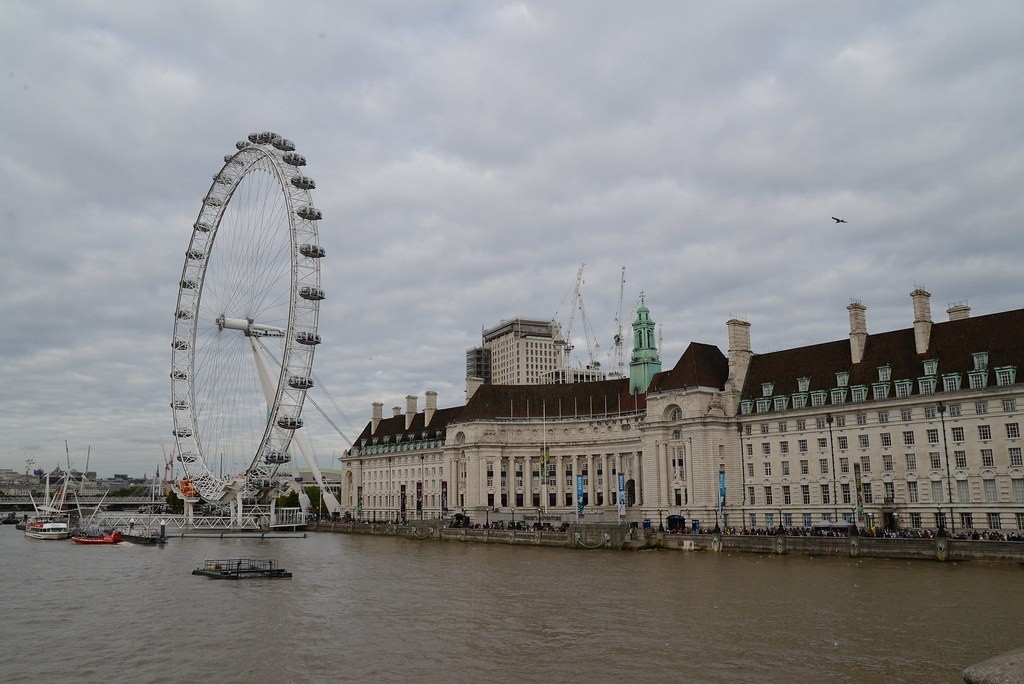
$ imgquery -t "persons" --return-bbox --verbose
[470,522,568,532]
[652,526,1024,542]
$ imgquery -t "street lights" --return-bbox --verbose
[775,506,785,533]
[658,507,665,531]
[849,505,860,536]
[713,507,721,533]
[461,508,542,530]
[935,503,947,537]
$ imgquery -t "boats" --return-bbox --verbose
[116,525,166,546]
[72,529,124,544]
[25,469,72,540]
